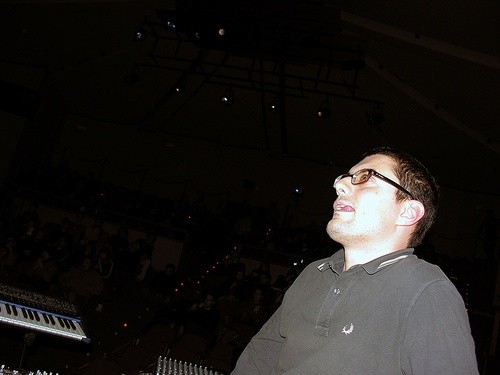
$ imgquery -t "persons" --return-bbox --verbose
[136,286,188,348]
[413,236,436,264]
[230,147,481,375]
[0,161,343,343]
[169,295,221,361]
[477,208,500,256]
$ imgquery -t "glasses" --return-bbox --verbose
[333,168,416,200]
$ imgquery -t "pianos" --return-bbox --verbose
[0,285,91,375]
